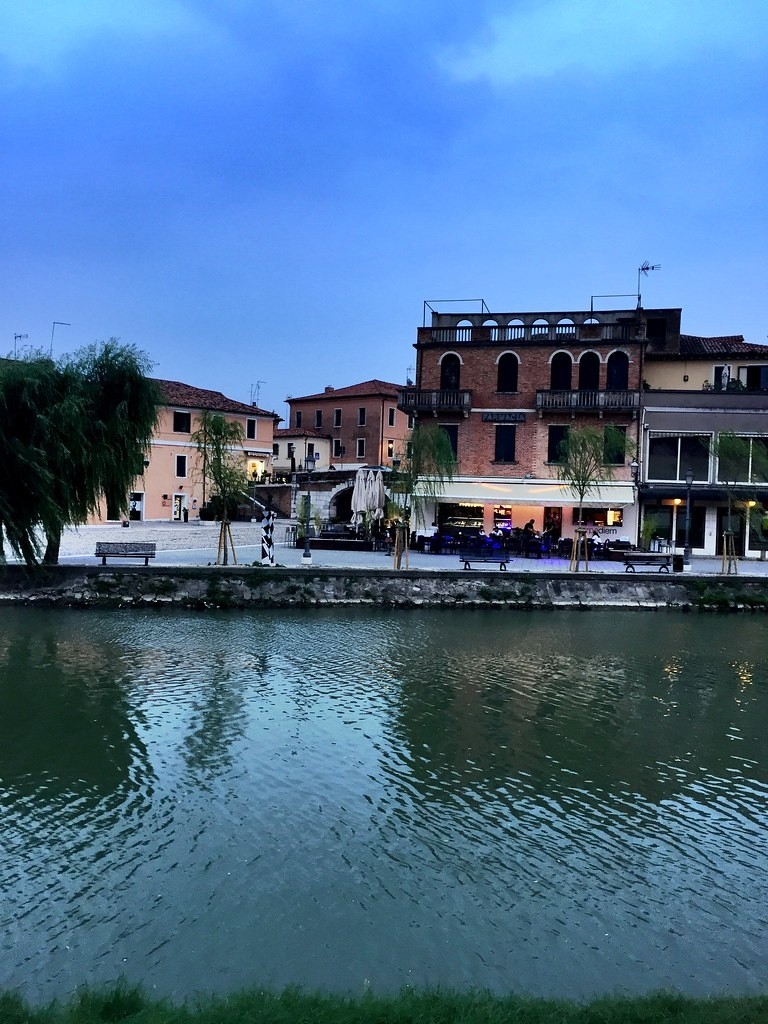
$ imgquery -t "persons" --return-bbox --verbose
[253,470,257,481]
[298,465,302,471]
[429,522,440,540]
[471,516,559,556]
[385,524,396,556]
[328,463,336,470]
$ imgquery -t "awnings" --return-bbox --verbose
[413,481,635,509]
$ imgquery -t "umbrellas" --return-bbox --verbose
[349,468,384,540]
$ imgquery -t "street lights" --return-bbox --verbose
[683,467,696,565]
[302,454,316,557]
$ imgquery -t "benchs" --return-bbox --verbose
[623,553,671,574]
[459,549,513,571]
[94,542,156,566]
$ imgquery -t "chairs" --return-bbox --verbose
[410,532,610,561]
